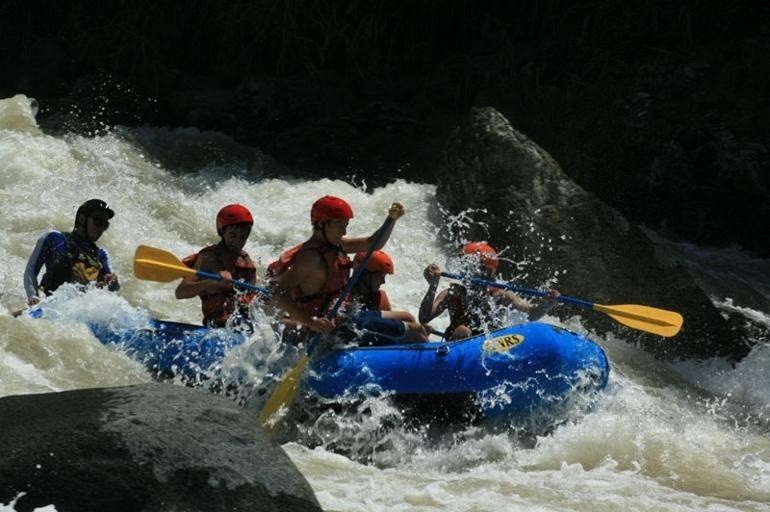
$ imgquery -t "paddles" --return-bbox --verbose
[258,209,398,433]
[134,246,271,294]
[433,269,684,337]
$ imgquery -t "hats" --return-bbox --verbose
[77,200,114,218]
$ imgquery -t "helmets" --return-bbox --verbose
[311,197,353,222]
[351,251,394,276]
[217,205,254,234]
[461,243,497,279]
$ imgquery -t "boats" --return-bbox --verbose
[14,297,611,481]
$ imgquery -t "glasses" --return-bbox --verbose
[89,217,111,230]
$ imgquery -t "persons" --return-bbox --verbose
[418,242,561,343]
[257,195,431,356]
[22,198,122,309]
[174,203,257,333]
